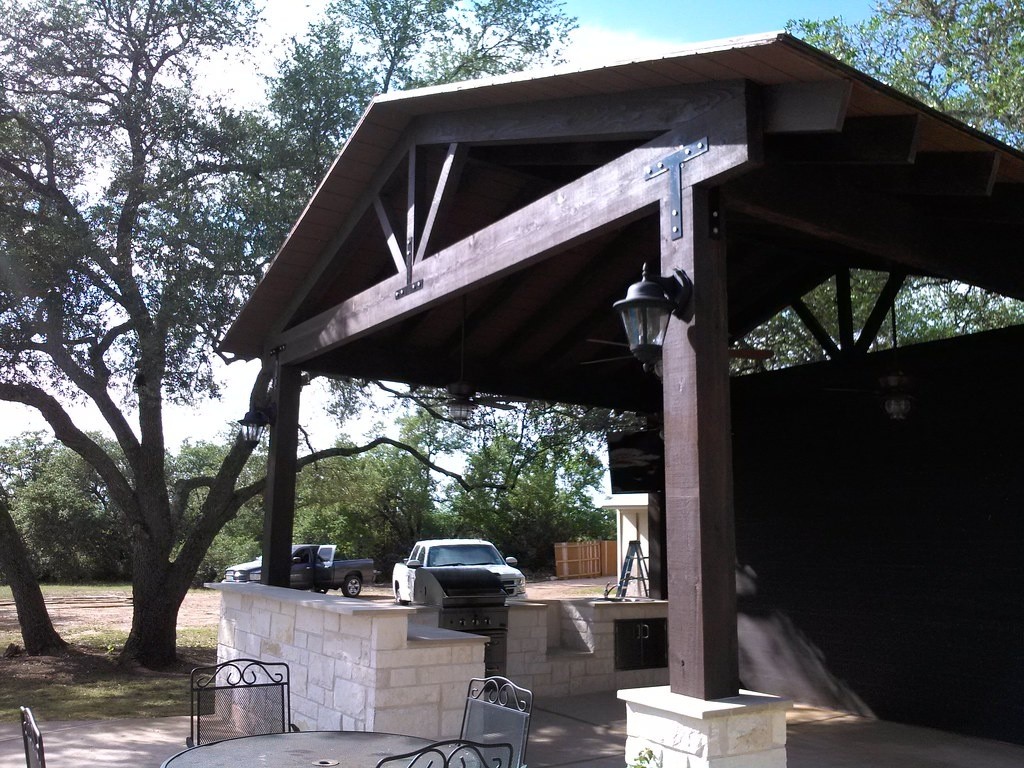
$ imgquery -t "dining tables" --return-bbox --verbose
[160,731,482,768]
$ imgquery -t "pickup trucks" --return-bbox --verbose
[222,545,375,598]
[392,538,528,608]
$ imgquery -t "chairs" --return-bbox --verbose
[186,659,300,748]
[21,706,46,768]
[377,739,513,768]
[459,676,533,768]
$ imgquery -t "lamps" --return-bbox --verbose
[237,403,276,447]
[612,262,691,372]
